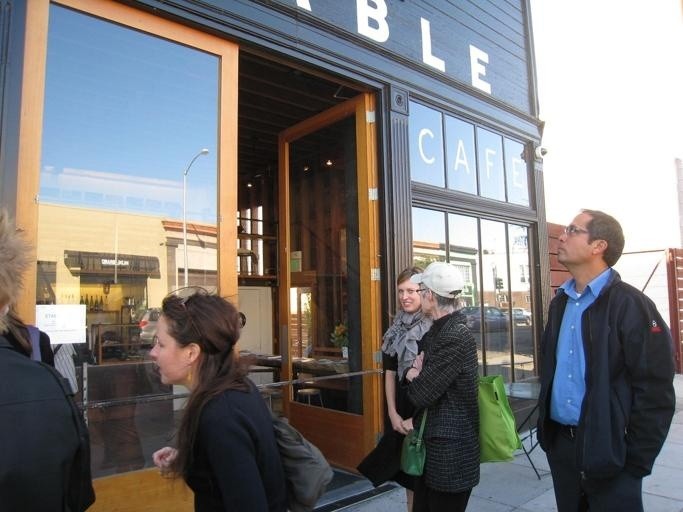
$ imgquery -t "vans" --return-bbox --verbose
[128,305,161,351]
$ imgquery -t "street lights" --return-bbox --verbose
[182,147,209,289]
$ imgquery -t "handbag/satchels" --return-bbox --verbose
[400,429,426,477]
[477,374,522,462]
[272,417,334,512]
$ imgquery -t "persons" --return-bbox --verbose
[535,208,676,512]
[0,212,97,512]
[402,259,485,511]
[376,267,432,512]
[141,289,289,512]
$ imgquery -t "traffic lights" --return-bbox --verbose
[494,277,504,290]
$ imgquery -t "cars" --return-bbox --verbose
[72,327,128,364]
[499,307,531,327]
[457,305,508,333]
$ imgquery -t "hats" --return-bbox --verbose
[410,262,464,298]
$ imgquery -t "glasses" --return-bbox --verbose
[178,297,202,338]
[416,288,427,294]
[564,226,588,233]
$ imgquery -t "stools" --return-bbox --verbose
[297,387,324,407]
